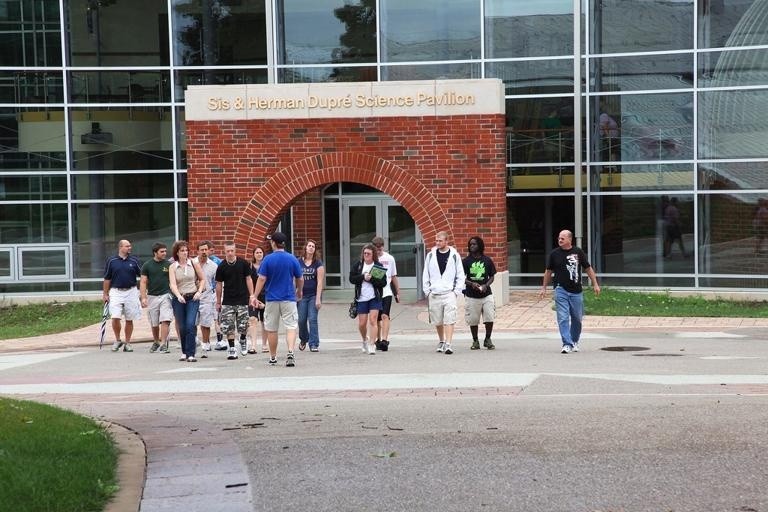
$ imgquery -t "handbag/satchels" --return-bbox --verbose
[349,301,358,319]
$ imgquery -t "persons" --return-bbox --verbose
[462,237,497,350]
[506,109,618,174]
[538,229,601,353]
[752,198,768,254]
[422,231,466,355]
[664,197,691,260]
[350,244,387,355]
[102,233,325,366]
[372,236,400,352]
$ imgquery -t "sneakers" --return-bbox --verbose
[471,341,480,350]
[159,344,170,353]
[176,337,269,362]
[310,345,318,352]
[484,338,495,349]
[123,343,133,352]
[561,342,579,353]
[269,355,278,364]
[436,342,453,354]
[299,342,306,351]
[150,343,160,352]
[112,340,122,352]
[362,336,389,355]
[286,351,296,366]
